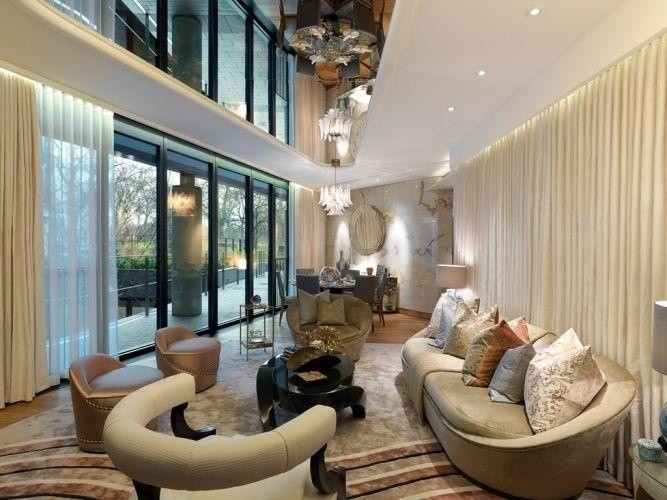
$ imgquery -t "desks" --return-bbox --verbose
[629,442,667,500]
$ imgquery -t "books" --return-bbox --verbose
[280,346,301,361]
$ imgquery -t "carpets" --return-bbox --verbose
[0,340,633,500]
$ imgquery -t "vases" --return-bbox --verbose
[336,250,350,275]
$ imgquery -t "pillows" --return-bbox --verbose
[423,288,606,435]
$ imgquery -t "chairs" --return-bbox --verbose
[100,372,347,500]
[286,294,373,362]
[154,326,222,394]
[68,352,164,454]
[275,264,388,327]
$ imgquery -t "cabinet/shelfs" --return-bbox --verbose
[371,277,397,314]
[239,304,274,361]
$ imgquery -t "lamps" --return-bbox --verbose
[289,13,378,217]
[651,299,667,457]
[435,264,468,290]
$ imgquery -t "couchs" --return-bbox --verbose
[401,326,638,500]
